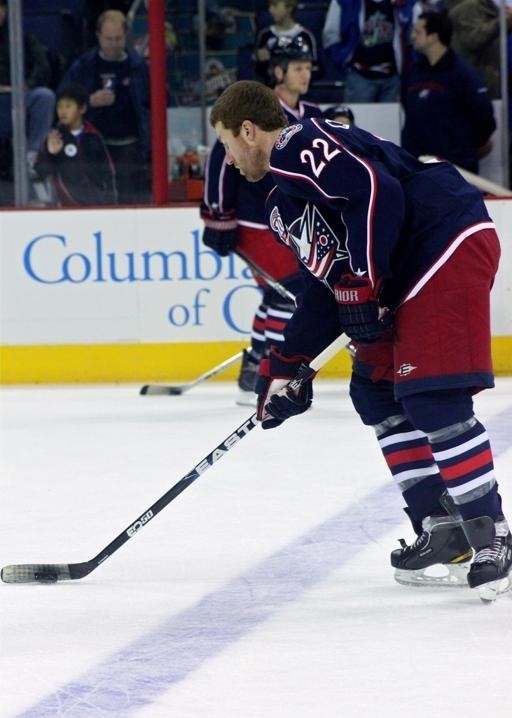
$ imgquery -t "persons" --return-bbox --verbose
[0,4,55,180]
[64,11,151,204]
[208,79,512,604]
[323,0,412,104]
[399,10,497,174]
[448,0,512,91]
[251,0,319,88]
[202,30,327,407]
[34,80,118,205]
[322,105,354,125]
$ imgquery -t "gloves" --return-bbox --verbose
[254,360,313,430]
[202,217,244,259]
[331,277,385,344]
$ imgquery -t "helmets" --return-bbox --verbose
[324,104,354,116]
[269,32,316,64]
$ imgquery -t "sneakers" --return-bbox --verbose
[462,514,512,589]
[391,516,472,571]
[239,355,262,394]
